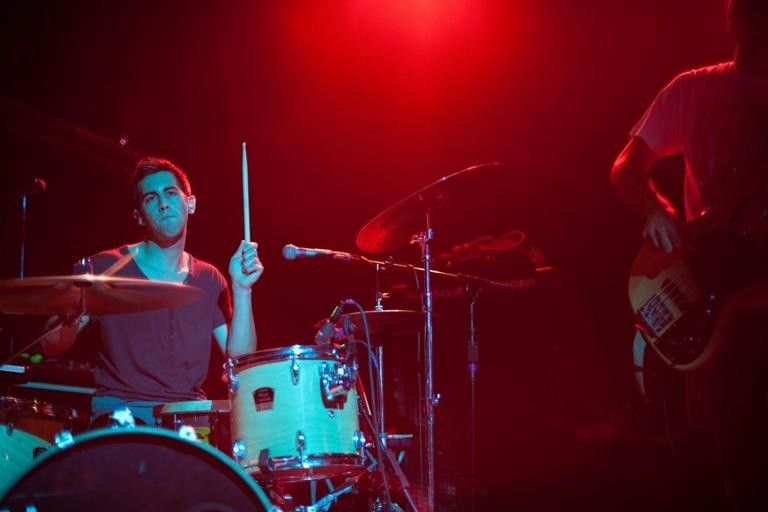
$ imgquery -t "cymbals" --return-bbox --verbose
[337,309,422,335]
[357,162,512,255]
[0,273,203,317]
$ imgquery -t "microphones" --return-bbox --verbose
[18,178,46,198]
[282,244,351,261]
[314,302,344,345]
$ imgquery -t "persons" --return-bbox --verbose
[40,157,267,441]
[608,3,764,494]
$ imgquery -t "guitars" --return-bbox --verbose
[628,182,768,370]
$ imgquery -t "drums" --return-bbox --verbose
[1,397,87,511]
[226,341,359,483]
[1,425,284,511]
[158,398,232,455]
